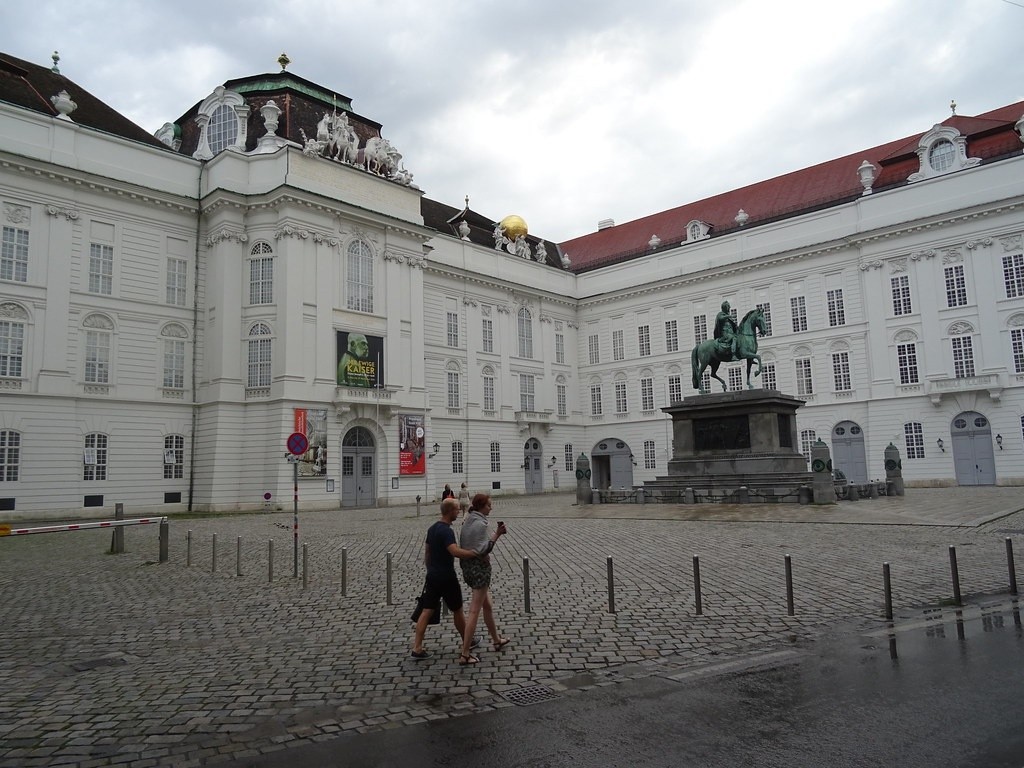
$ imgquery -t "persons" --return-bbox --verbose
[492,224,548,265]
[441,482,471,525]
[337,110,349,141]
[714,301,740,361]
[315,442,327,464]
[458,493,512,666]
[408,496,482,659]
[336,332,374,388]
[407,436,425,466]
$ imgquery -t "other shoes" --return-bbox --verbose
[410,649,433,659]
[468,636,483,649]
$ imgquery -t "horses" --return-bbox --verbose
[691,308,768,393]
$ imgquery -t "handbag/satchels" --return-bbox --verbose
[447,489,453,499]
[411,580,441,625]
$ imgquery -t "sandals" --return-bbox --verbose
[459,654,479,664]
[493,637,511,651]
